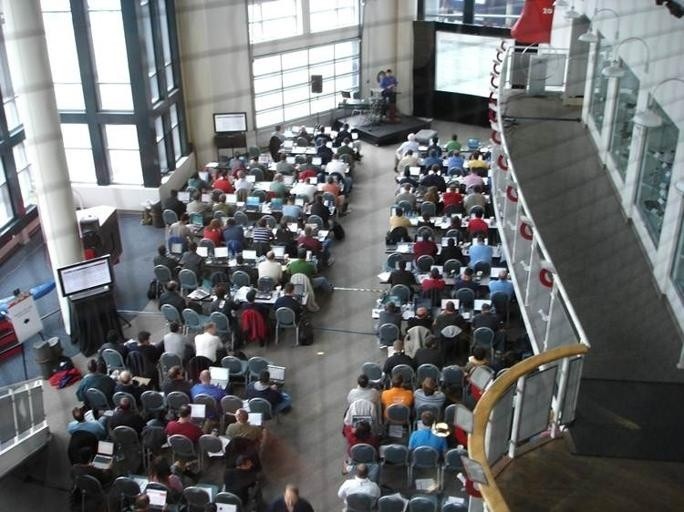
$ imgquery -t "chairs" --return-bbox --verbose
[66,127,357,510]
[344,133,532,512]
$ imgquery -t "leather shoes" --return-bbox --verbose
[342,460,349,475]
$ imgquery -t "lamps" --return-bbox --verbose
[552,0,684,129]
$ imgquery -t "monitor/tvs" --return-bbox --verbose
[213,112,247,134]
[56,255,116,297]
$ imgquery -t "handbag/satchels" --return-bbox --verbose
[148,278,161,299]
[333,224,344,239]
[300,320,313,345]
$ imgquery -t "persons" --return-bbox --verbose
[155,189,334,335]
[377,259,522,384]
[189,151,352,215]
[271,120,363,175]
[381,69,399,115]
[389,132,506,265]
[338,375,467,511]
[68,321,290,511]
[272,484,313,511]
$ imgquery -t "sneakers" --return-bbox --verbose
[192,470,203,483]
[328,284,334,292]
[342,210,352,215]
[328,257,335,266]
